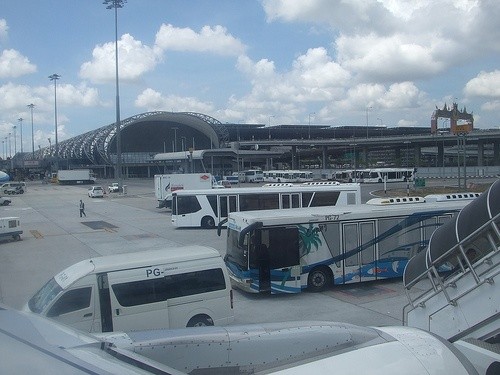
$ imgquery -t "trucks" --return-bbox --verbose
[58,169,96,185]
[153,172,225,208]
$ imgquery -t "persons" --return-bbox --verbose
[453,248,478,275]
[79,200,87,218]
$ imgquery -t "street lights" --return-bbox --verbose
[102,0,128,193]
[366,106,373,139]
[308,111,316,140]
[27,103,36,163]
[268,115,276,141]
[376,118,383,138]
[48,73,61,145]
[17,118,25,165]
[1,125,18,169]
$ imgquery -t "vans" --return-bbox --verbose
[87,185,104,198]
[19,244,236,334]
[0,182,27,195]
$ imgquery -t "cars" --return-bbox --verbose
[108,183,119,192]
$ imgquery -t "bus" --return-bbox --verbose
[333,167,417,183]
[218,170,314,188]
[160,180,362,230]
[216,192,486,297]
